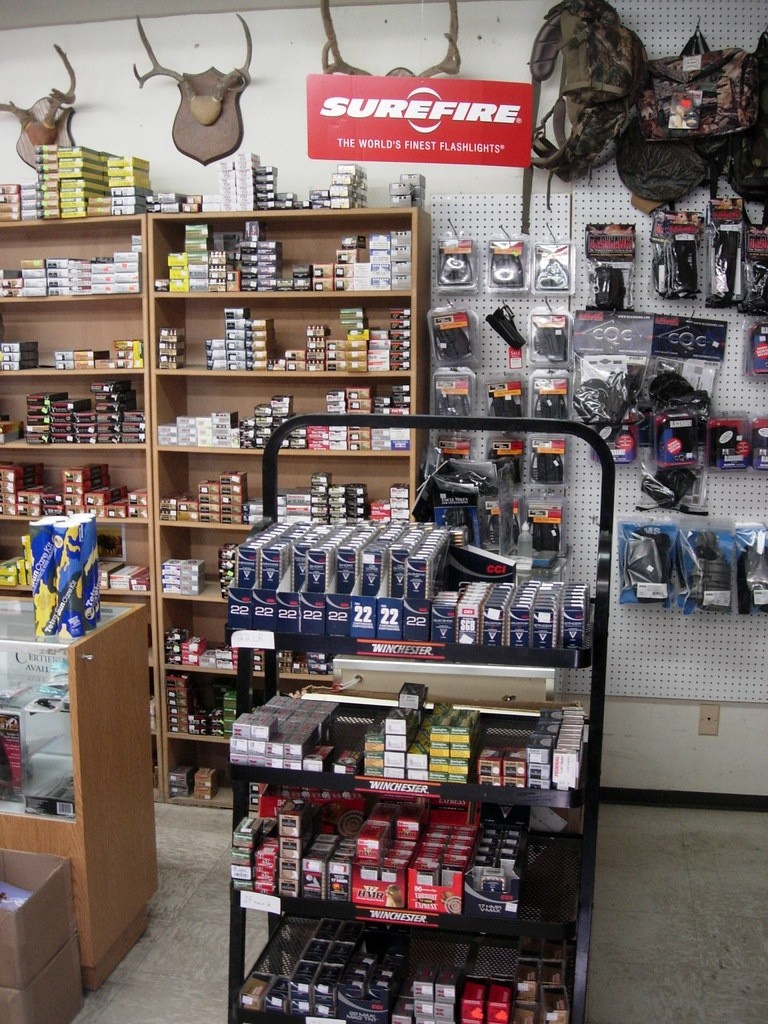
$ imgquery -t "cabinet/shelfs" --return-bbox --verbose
[149,207,433,810]
[0,209,165,803]
[225,412,617,1024]
[0,596,160,991]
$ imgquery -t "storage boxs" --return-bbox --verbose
[153,220,411,293]
[0,534,33,586]
[218,519,589,651]
[334,683,586,792]
[163,628,334,674]
[160,559,204,595]
[55,340,143,371]
[0,341,40,371]
[232,777,533,918]
[161,471,409,524]
[229,691,337,772]
[0,235,144,297]
[205,305,413,372]
[158,382,412,451]
[234,912,571,1024]
[0,145,369,220]
[390,173,427,208]
[25,774,74,815]
[0,380,145,443]
[167,673,238,739]
[157,327,185,370]
[0,849,86,1024]
[98,561,150,592]
[0,461,148,518]
